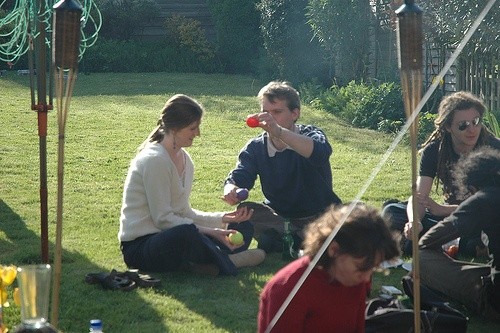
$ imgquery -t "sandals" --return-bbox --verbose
[85,267,162,292]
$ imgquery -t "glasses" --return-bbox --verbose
[458,116,480,131]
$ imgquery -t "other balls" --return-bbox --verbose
[237,187,249,200]
[229,231,243,245]
[247,115,259,128]
[448,245,458,254]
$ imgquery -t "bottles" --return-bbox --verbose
[88,319,104,333]
[282,219,295,260]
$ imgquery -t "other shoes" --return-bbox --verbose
[257,228,301,253]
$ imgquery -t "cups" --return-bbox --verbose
[16,264,52,329]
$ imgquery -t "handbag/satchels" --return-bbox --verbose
[364,275,467,333]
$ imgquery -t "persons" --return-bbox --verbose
[383,91,500,322]
[118,94,267,274]
[223,81,344,257]
[258,202,402,333]
